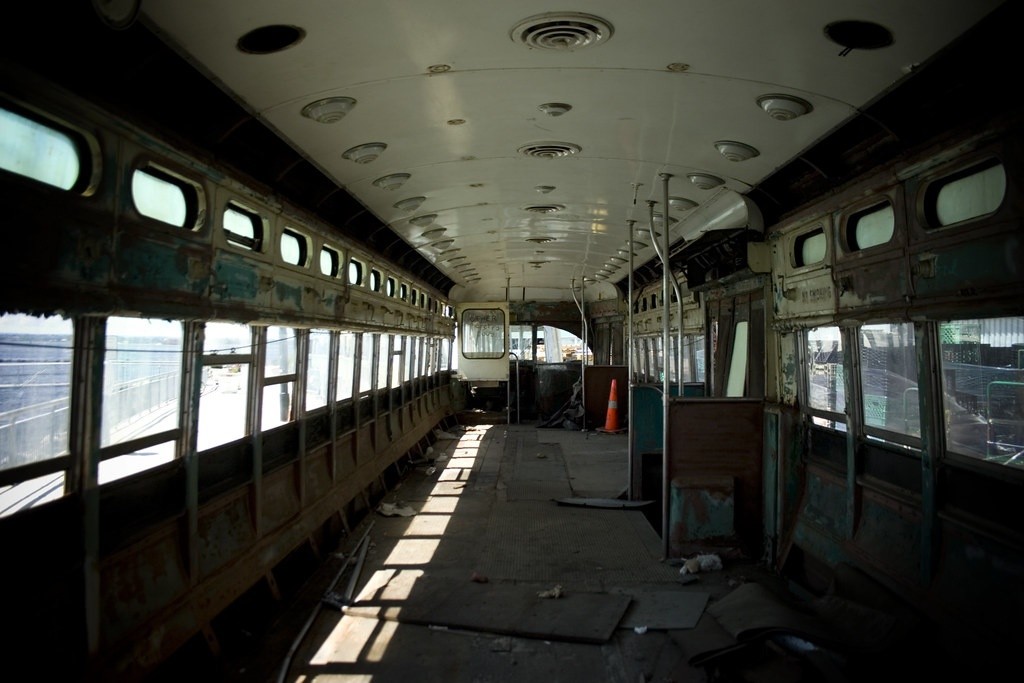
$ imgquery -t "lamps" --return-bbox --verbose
[668,196,699,211]
[715,139,761,163]
[373,172,411,192]
[342,142,386,164]
[392,196,479,284]
[755,92,812,122]
[573,214,679,292]
[301,93,355,124]
[685,173,726,190]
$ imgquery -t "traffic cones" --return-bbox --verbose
[594,379,628,433]
